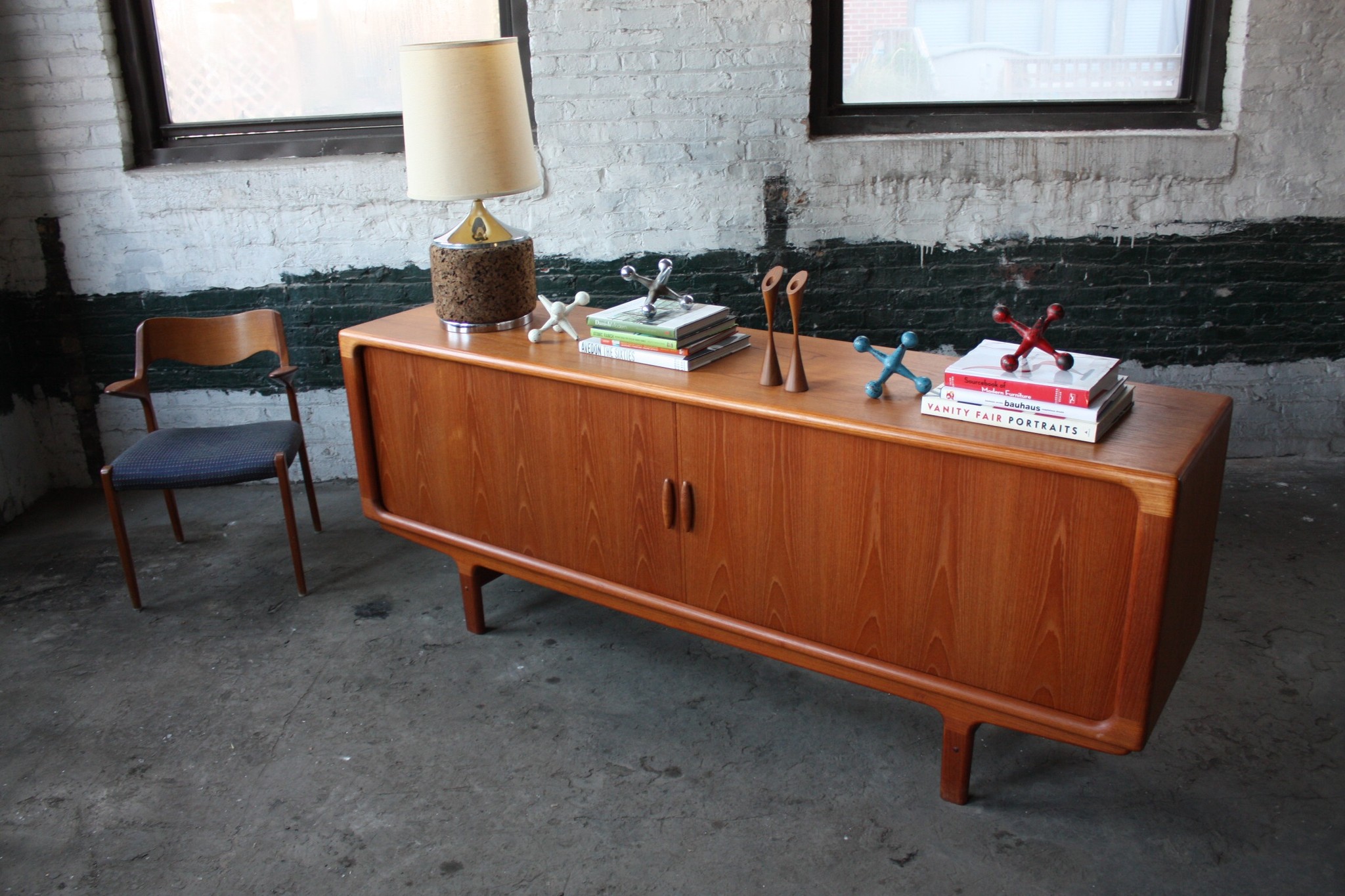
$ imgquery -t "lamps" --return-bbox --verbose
[400,35,543,331]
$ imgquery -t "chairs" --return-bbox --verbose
[99,307,322,605]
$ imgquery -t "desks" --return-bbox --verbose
[340,306,1234,803]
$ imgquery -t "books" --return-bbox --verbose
[921,338,1134,443]
[579,295,751,373]
